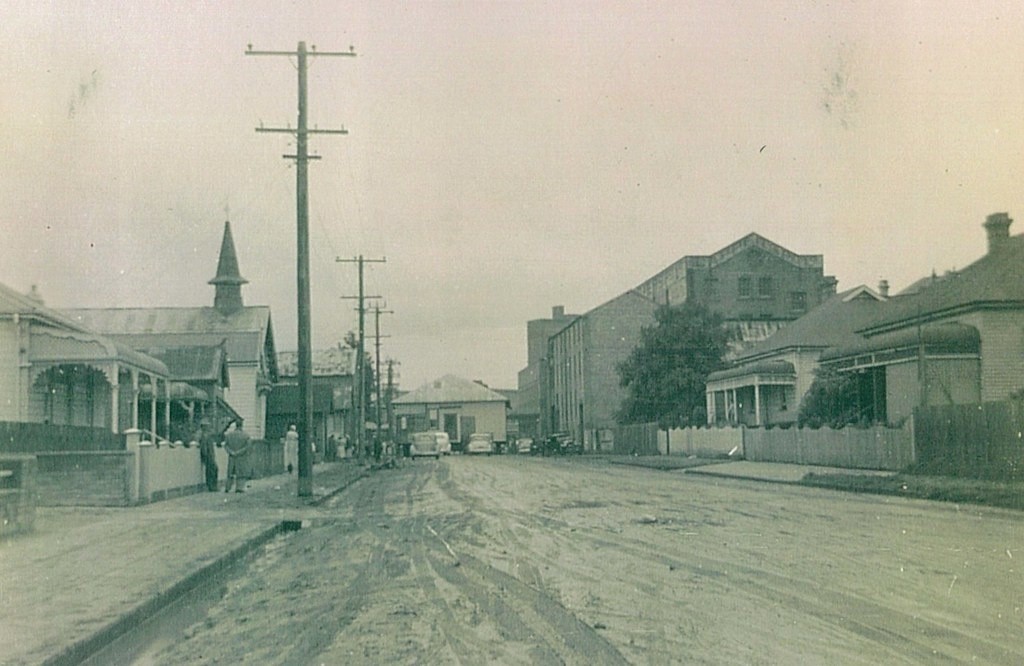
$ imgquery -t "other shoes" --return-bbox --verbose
[224,489,229,494]
[208,486,220,491]
[235,490,245,494]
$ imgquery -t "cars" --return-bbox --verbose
[516,432,583,455]
[434,430,452,455]
[410,432,440,459]
[463,437,492,456]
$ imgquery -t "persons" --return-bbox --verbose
[284,424,298,474]
[531,434,558,456]
[381,439,415,468]
[194,418,221,492]
[224,419,251,493]
[357,428,381,460]
[329,432,353,463]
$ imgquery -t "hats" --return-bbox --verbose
[199,418,210,425]
